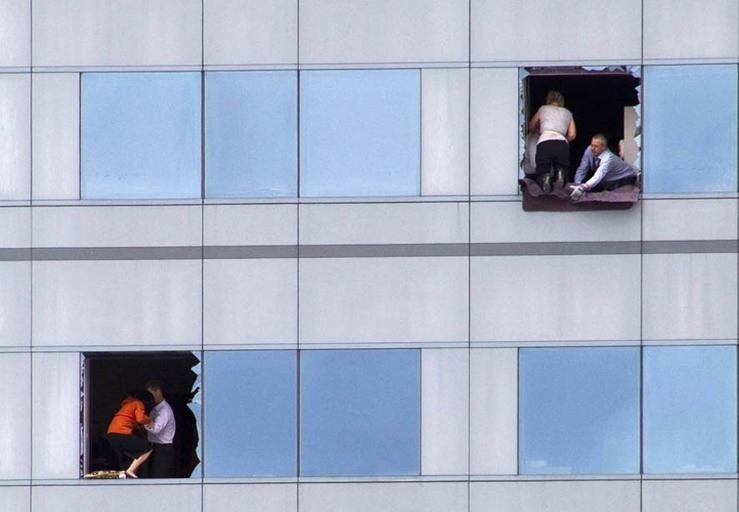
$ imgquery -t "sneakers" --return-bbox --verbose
[542,168,564,193]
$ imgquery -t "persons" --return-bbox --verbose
[525,90,579,194]
[106,381,176,478]
[568,133,637,200]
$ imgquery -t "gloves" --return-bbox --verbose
[569,184,584,201]
